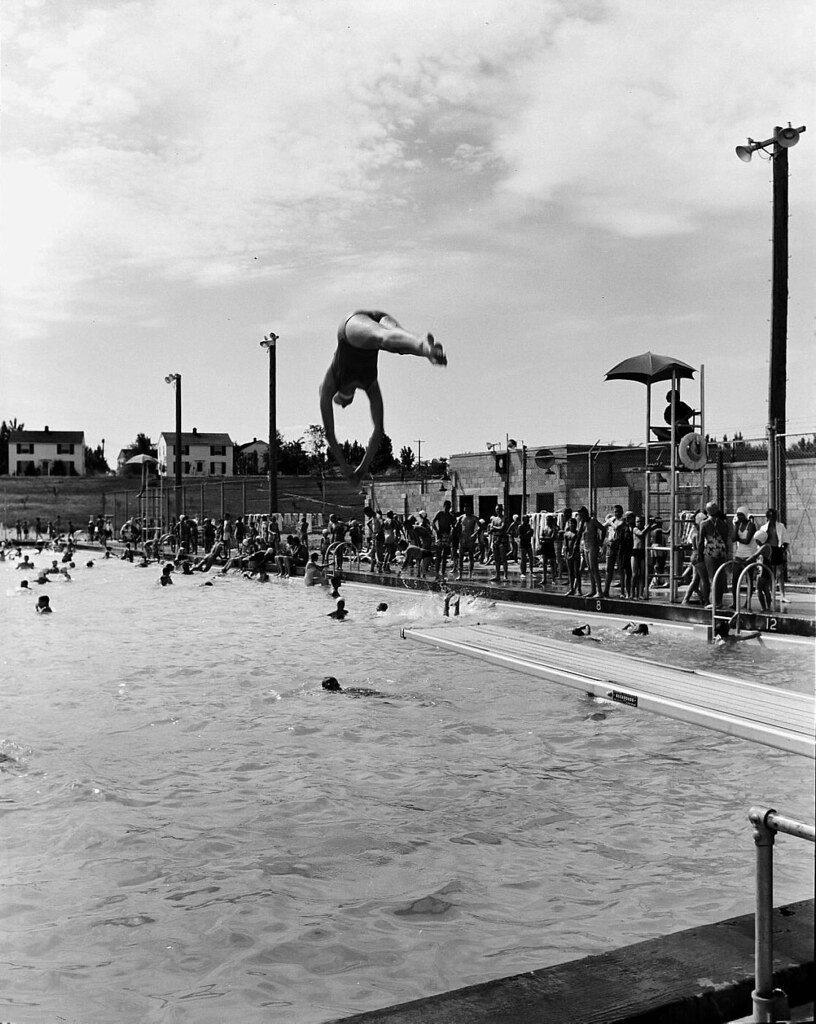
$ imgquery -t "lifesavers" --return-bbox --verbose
[678,432,709,470]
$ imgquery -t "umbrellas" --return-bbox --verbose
[124,454,160,464]
[604,350,696,386]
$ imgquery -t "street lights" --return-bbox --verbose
[737,126,805,584]
[164,373,183,526]
[258,332,278,517]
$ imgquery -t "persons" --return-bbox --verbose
[0,390,788,692]
[319,310,448,485]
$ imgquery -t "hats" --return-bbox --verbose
[490,516,495,520]
[695,512,707,525]
[512,514,519,522]
[737,506,748,519]
[419,510,426,518]
[322,529,328,534]
[479,519,485,525]
[179,515,185,521]
[706,501,717,515]
